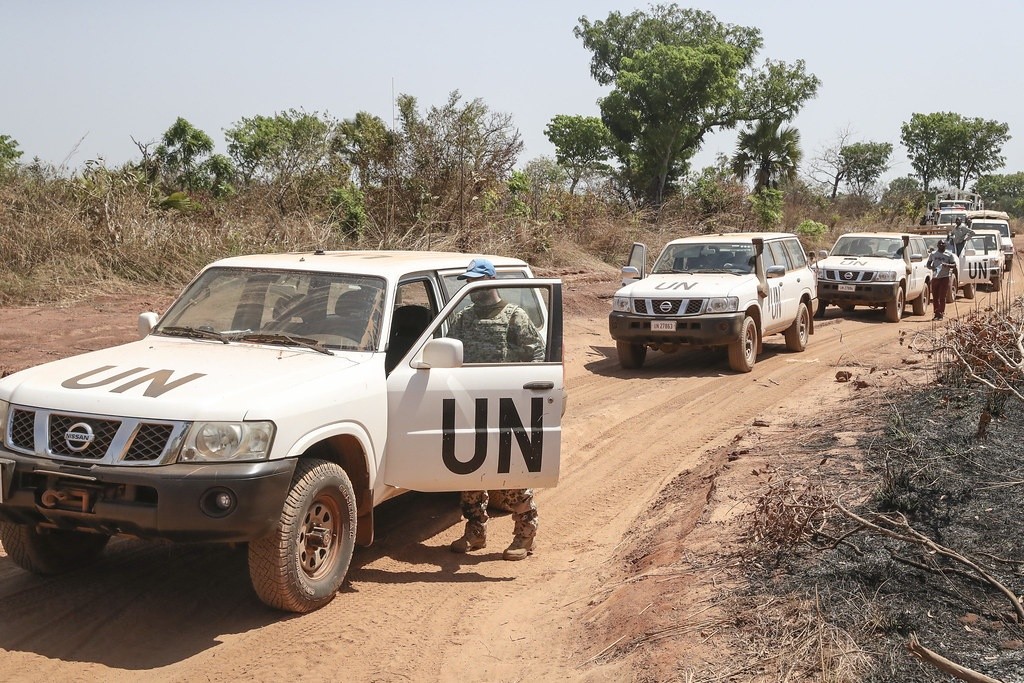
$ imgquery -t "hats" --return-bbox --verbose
[457,259,496,280]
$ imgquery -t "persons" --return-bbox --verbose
[969,187,979,201]
[445,258,545,560]
[945,194,952,200]
[949,216,976,257]
[932,208,940,225]
[966,219,972,228]
[926,240,956,319]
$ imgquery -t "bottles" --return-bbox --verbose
[932,264,943,278]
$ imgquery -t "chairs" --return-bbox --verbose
[975,236,995,249]
[853,244,873,256]
[265,294,324,338]
[381,304,432,372]
[887,244,900,253]
[697,246,752,267]
[323,289,383,344]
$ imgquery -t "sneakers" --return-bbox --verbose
[451,524,487,552]
[503,534,535,561]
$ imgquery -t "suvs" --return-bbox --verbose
[609,232,819,373]
[810,232,933,323]
[923,189,1014,304]
[0,248,568,613]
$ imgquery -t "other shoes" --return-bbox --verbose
[932,316,938,320]
[938,317,943,321]
